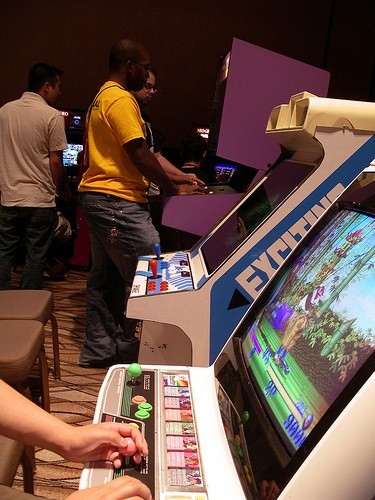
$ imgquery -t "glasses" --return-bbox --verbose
[145,83,156,90]
[131,59,151,70]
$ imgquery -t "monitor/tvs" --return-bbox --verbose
[61,144,83,167]
[239,204,375,460]
[198,158,318,278]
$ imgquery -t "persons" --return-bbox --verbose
[49,182,72,273]
[0,379,153,500]
[131,70,197,198]
[0,62,68,291]
[76,41,206,368]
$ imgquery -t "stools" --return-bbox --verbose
[0,289,67,493]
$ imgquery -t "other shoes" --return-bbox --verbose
[92,354,126,369]
